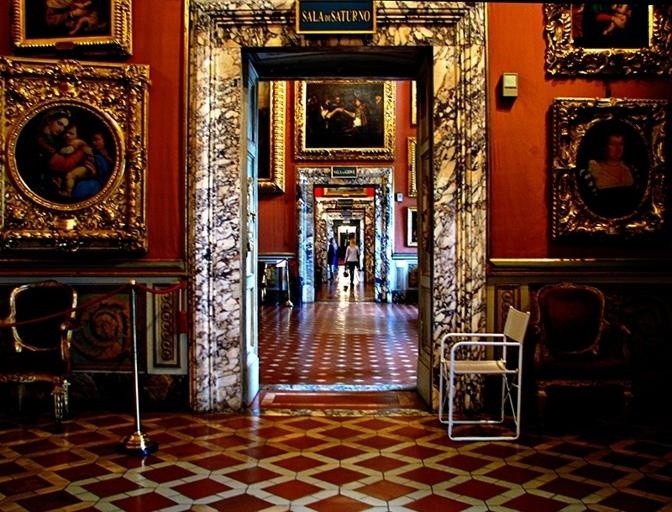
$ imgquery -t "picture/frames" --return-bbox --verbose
[8,0,134,55]
[406,137,417,198]
[540,3,670,81]
[549,98,669,249]
[293,81,397,162]
[258,81,286,194]
[404,205,419,248]
[0,53,150,255]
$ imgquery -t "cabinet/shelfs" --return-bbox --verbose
[391,252,419,304]
[261,252,297,309]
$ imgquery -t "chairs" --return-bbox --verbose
[527,281,635,438]
[1,279,78,434]
[440,304,531,441]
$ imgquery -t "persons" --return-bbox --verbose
[45,0,101,38]
[585,132,631,186]
[32,111,110,203]
[583,1,631,47]
[328,238,338,281]
[344,238,362,287]
[308,95,383,146]
